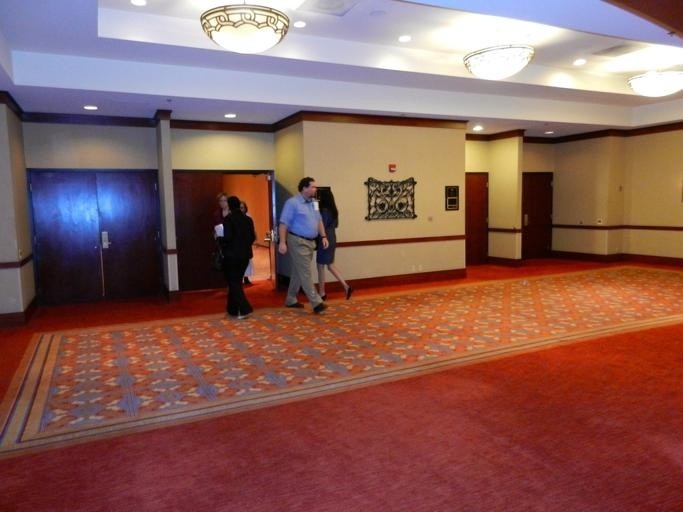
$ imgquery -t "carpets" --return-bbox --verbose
[1,264,682,457]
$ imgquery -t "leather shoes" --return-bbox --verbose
[285,287,352,312]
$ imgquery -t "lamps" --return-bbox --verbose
[627,66,682,100]
[459,42,534,84]
[196,2,291,57]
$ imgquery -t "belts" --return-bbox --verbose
[290,232,318,240]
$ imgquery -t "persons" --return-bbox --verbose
[314,189,352,301]
[216,196,256,320]
[238,201,256,284]
[216,191,231,221]
[277,176,330,314]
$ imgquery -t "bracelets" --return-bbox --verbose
[320,236,327,238]
